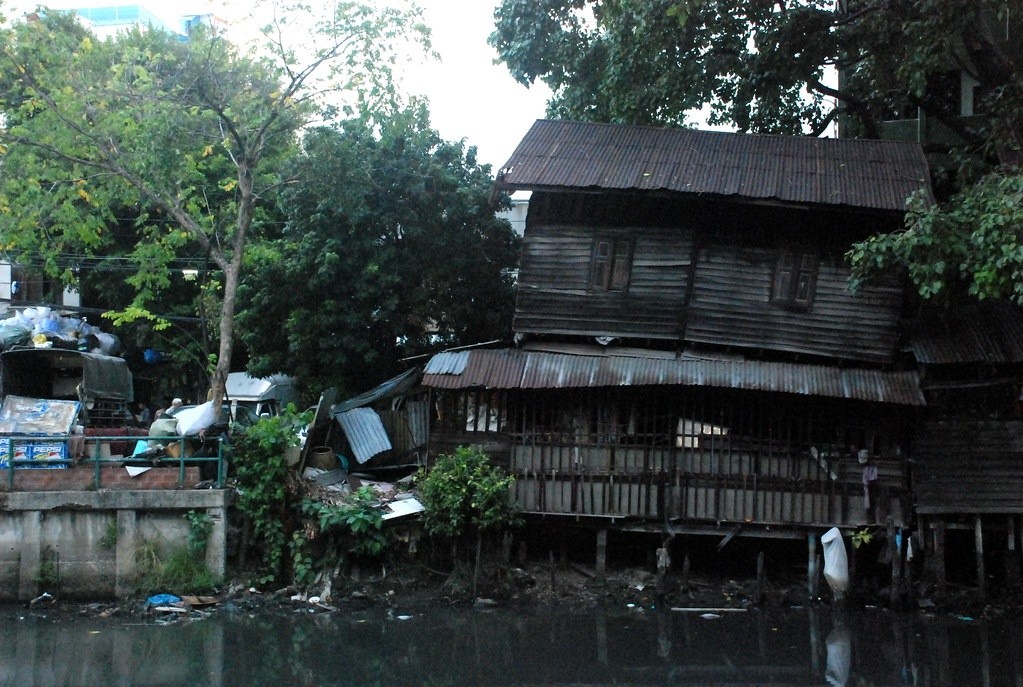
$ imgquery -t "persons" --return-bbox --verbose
[135,397,184,430]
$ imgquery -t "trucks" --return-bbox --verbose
[215,371,310,432]
